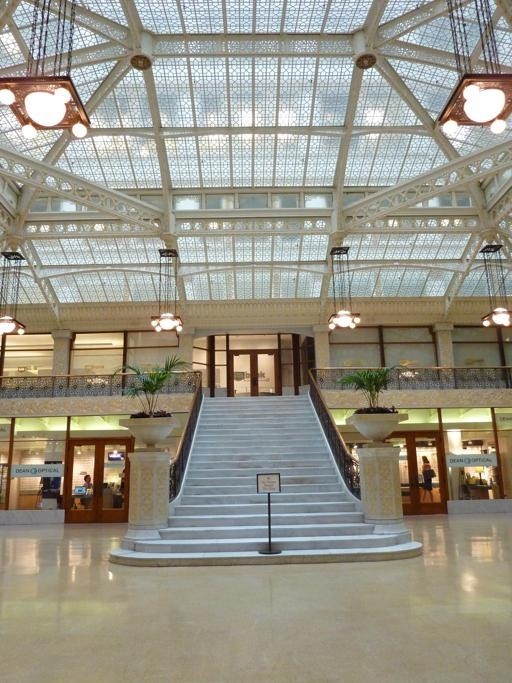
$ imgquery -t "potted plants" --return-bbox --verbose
[337,366,414,441]
[112,354,193,446]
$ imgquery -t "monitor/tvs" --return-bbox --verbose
[108,452,124,460]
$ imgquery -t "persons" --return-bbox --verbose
[422,456,434,501]
[79,474,93,504]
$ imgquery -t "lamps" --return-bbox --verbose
[475,466,485,486]
[150,249,183,332]
[328,245,360,329]
[0,1,91,139]
[478,245,512,327]
[435,0,512,135]
[0,252,26,335]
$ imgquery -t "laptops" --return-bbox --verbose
[72,486,87,496]
